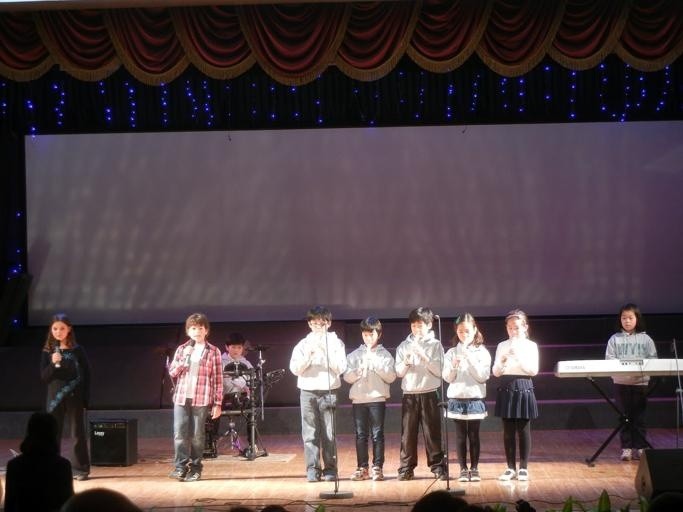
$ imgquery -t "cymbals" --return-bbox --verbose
[246,345,267,351]
[224,362,247,377]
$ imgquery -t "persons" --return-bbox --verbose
[203,333,266,458]
[41,314,90,481]
[411,491,536,512]
[394,307,447,480]
[1,412,144,512]
[605,303,657,461]
[442,312,492,481]
[168,313,223,481]
[290,306,347,481]
[492,308,539,481]
[343,316,396,481]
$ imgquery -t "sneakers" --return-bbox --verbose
[324,471,337,482]
[398,468,414,481]
[306,471,320,482]
[470,468,481,482]
[499,468,517,481]
[73,466,90,481]
[458,468,470,482]
[168,464,202,483]
[351,467,368,481]
[620,447,650,458]
[372,466,383,481]
[435,468,448,480]
[517,468,529,480]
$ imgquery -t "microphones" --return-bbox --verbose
[321,320,328,327]
[184,338,196,370]
[52,336,61,369]
[434,313,441,322]
[669,338,678,353]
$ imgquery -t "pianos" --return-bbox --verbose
[554,358,683,379]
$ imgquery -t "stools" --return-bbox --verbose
[211,408,249,460]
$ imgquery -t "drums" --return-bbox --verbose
[222,391,248,412]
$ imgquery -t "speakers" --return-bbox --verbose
[633,448,683,512]
[87,418,138,466]
